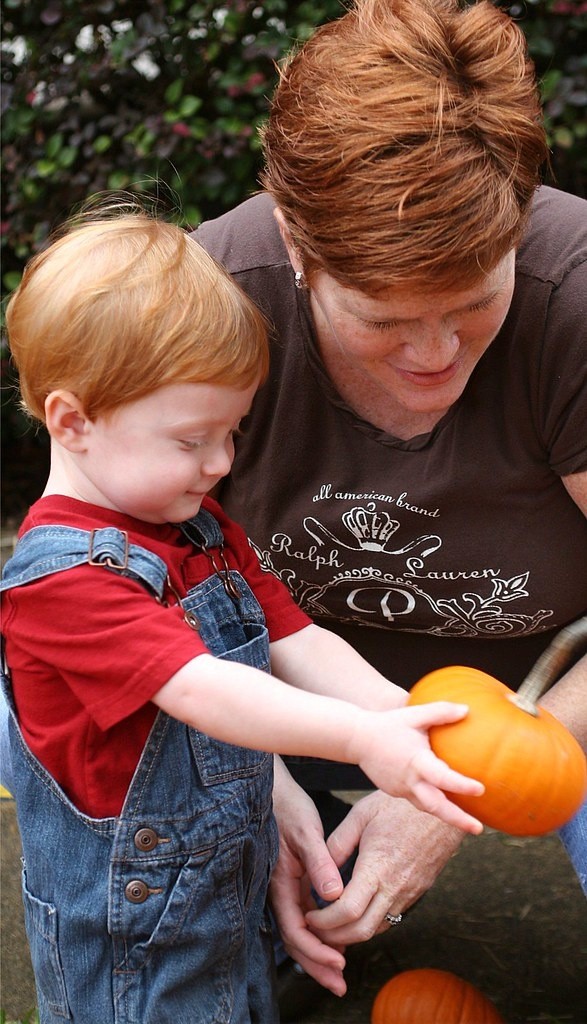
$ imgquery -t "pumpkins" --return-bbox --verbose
[406,665,587,838]
[370,968,503,1024]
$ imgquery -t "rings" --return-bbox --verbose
[384,914,402,924]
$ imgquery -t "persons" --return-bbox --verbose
[1,218,486,1024]
[193,0,587,1024]
